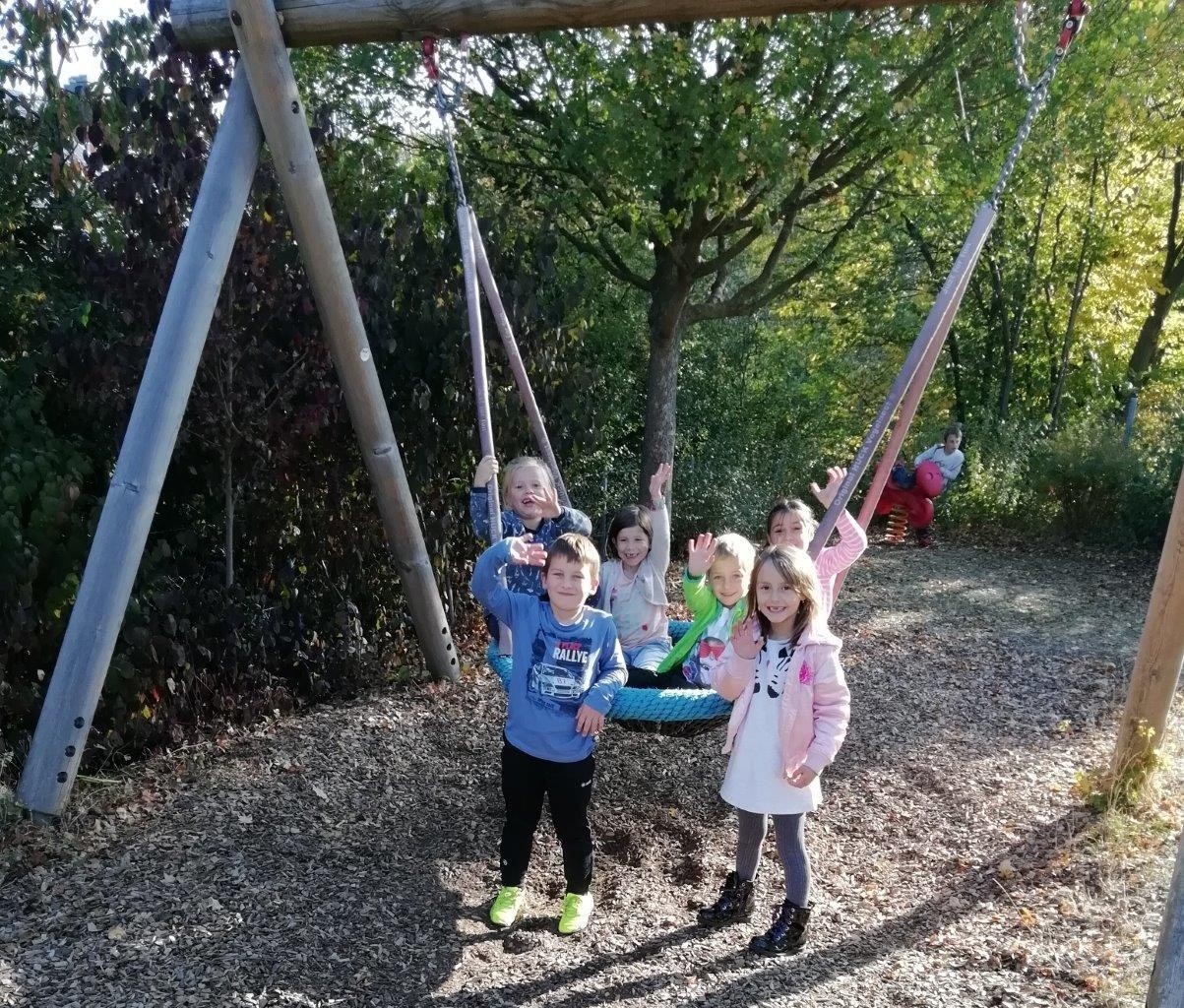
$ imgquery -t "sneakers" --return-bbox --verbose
[559,892,596,933]
[489,886,527,926]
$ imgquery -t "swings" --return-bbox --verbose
[416,1,1098,740]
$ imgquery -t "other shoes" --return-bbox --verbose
[917,532,928,547]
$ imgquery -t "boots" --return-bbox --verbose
[748,900,815,958]
[696,871,759,926]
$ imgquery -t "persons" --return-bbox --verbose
[914,429,964,547]
[469,455,870,953]
